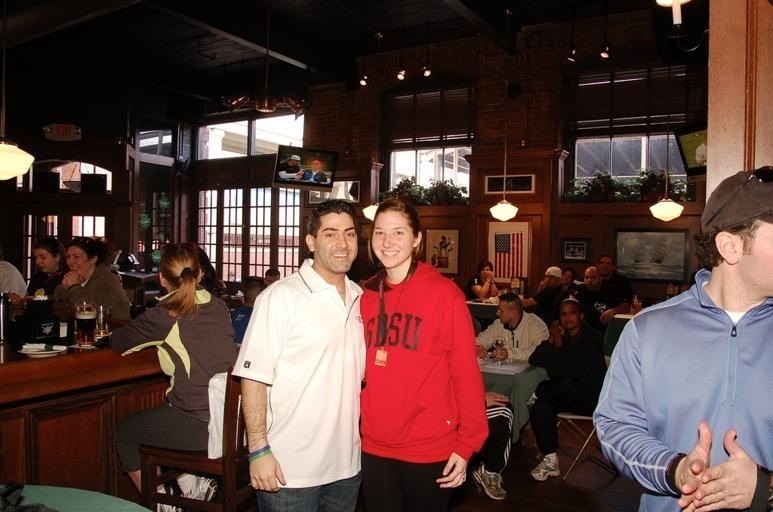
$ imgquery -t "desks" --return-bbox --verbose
[0,476,148,511]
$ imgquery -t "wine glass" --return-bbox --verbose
[491,336,506,366]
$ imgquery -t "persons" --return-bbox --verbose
[592,166,773,512]
[464,254,632,503]
[0,236,140,321]
[278,155,304,180]
[231,267,280,344]
[303,160,321,180]
[184,240,216,290]
[354,198,489,512]
[231,199,365,511]
[109,243,238,512]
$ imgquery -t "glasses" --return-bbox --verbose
[707,164,771,224]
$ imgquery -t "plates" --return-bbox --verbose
[16,349,66,358]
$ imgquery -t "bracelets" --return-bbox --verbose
[249,445,272,463]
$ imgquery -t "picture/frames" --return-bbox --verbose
[560,236,592,263]
[299,176,362,205]
[611,227,690,285]
[485,217,533,290]
[424,228,461,277]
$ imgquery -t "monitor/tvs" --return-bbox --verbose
[271,145,339,192]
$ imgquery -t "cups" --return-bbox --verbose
[631,298,641,314]
[71,301,98,348]
[93,305,113,337]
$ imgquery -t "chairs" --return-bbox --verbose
[130,360,257,510]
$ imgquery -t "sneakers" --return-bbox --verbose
[530,456,562,481]
[156,474,217,511]
[470,462,506,502]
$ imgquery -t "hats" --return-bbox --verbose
[289,155,302,163]
[545,266,562,278]
[312,159,322,167]
[699,167,771,234]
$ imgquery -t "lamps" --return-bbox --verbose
[595,1,612,61]
[0,132,38,186]
[418,65,433,79]
[357,30,372,86]
[483,122,527,224]
[564,1,579,64]
[220,20,304,122]
[638,98,686,223]
[362,152,381,223]
